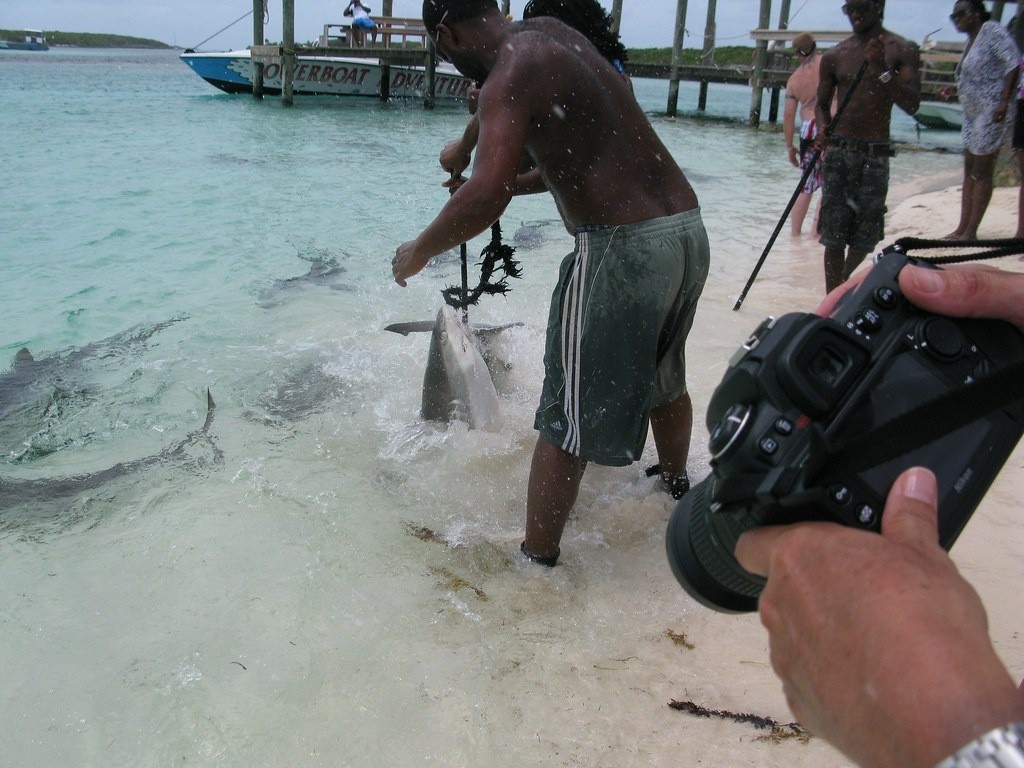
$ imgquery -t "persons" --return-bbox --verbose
[391,0,711,571]
[343,0,379,49]
[810,0,922,295]
[657,254,1024,767]
[935,1,1024,241]
[783,33,838,238]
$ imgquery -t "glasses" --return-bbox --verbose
[842,0,877,15]
[435,11,453,63]
[951,7,965,19]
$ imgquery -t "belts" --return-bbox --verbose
[828,139,895,157]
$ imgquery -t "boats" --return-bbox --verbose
[915,103,962,129]
[0,29,51,51]
[178,51,471,102]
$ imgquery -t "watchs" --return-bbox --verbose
[941,724,1022,768]
[875,67,896,83]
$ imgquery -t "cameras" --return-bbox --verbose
[665,255,1020,616]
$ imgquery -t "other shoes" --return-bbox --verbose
[520,540,560,567]
[646,465,689,500]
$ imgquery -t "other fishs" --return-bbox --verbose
[384,304,525,434]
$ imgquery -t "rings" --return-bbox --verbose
[996,116,1003,120]
